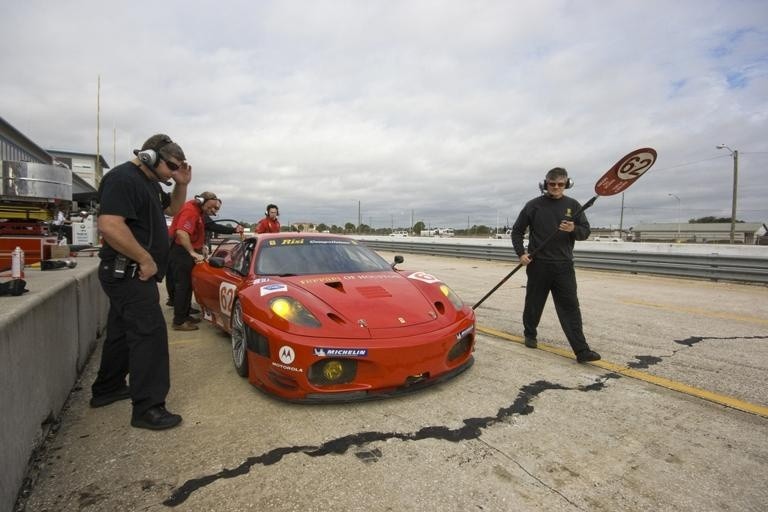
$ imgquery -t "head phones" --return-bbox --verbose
[539,178,574,190]
[133,138,172,168]
[195,195,216,206]
[265,205,279,216]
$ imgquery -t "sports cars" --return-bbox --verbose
[190,232,476,404]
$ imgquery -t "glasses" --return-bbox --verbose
[548,182,566,188]
[160,155,180,171]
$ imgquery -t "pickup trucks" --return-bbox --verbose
[495,229,511,239]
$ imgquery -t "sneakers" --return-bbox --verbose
[574,346,601,362]
[187,316,202,323]
[89,385,132,408]
[130,404,183,431]
[172,320,199,331]
[523,335,539,348]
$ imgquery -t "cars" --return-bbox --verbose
[594,235,622,241]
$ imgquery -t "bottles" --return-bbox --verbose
[11,246,25,279]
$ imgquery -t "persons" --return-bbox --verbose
[254,204,280,233]
[168,191,217,331]
[511,167,601,363]
[165,198,244,314]
[90,134,192,431]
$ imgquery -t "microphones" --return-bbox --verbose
[542,189,554,197]
[149,168,172,186]
[275,215,279,220]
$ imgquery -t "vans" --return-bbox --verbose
[420,226,454,237]
[389,230,407,237]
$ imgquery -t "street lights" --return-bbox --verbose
[667,190,682,245]
[715,142,741,243]
